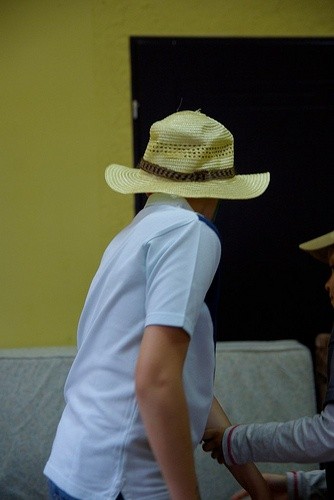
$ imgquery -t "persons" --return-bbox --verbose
[199,231,334,500]
[43,109,272,500]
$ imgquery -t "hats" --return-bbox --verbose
[104,110,271,199]
[299,231,334,263]
